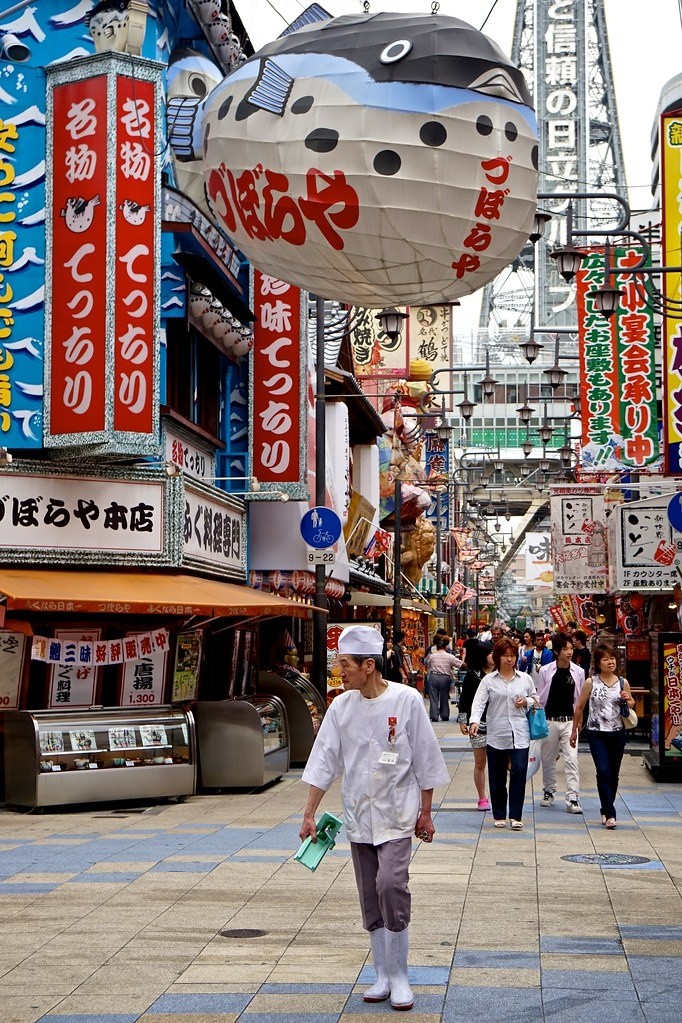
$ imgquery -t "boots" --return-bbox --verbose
[363,928,392,1003]
[384,923,414,1010]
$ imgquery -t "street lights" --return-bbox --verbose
[529,193,682,319]
[516,381,583,464]
[519,311,580,413]
[519,421,579,474]
[436,442,515,632]
[314,295,409,706]
[395,352,500,631]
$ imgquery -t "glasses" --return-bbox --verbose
[287,654,298,657]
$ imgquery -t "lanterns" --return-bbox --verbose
[250,571,345,600]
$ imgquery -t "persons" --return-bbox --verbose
[568,640,635,828]
[386,632,408,684]
[537,631,585,813]
[457,642,495,811]
[423,621,592,722]
[468,638,540,828]
[299,625,451,1010]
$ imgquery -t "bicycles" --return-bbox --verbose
[451,669,464,723]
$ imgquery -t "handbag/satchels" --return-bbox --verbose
[527,697,549,740]
[619,676,638,729]
[526,738,542,782]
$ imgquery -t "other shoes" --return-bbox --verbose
[601,815,607,824]
[441,715,449,721]
[606,819,617,828]
[432,718,438,722]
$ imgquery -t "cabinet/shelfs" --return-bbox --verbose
[189,693,291,788]
[255,666,327,762]
[0,704,198,808]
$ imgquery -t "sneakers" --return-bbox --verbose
[566,802,583,814]
[540,792,554,806]
[477,797,491,810]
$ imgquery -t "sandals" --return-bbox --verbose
[494,819,506,828]
[509,819,523,828]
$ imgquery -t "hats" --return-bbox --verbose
[338,625,384,654]
[281,628,297,650]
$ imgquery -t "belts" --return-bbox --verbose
[547,716,572,722]
[431,671,444,674]
